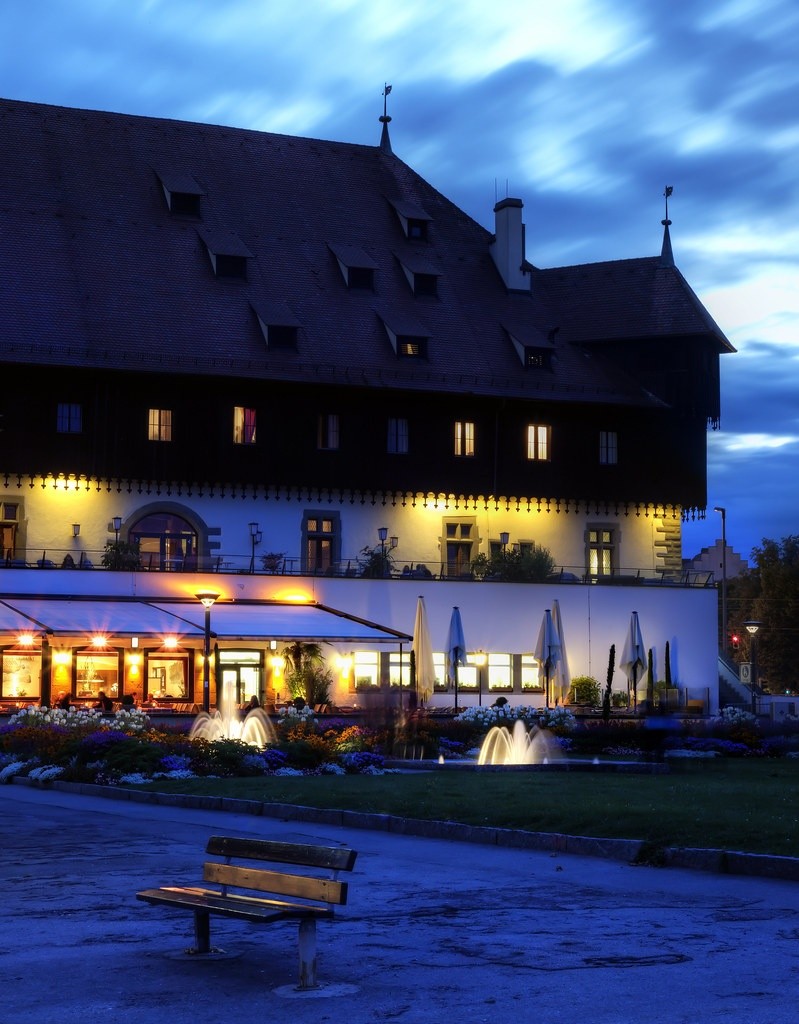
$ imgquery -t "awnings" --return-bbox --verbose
[0,600,411,645]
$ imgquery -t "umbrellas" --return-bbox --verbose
[538,597,570,708]
[534,607,562,715]
[413,594,434,709]
[442,606,467,714]
[620,610,648,714]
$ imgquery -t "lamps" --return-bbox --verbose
[70,522,81,537]
[270,641,276,650]
[389,537,399,547]
[511,543,519,552]
[248,521,259,536]
[378,527,389,541]
[112,516,121,529]
[254,530,262,544]
[500,532,510,544]
[132,637,139,648]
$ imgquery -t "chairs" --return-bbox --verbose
[407,706,467,721]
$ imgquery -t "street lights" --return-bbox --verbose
[472,650,487,706]
[742,621,764,714]
[713,505,728,655]
[195,591,221,713]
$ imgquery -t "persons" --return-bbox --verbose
[144,695,158,706]
[91,691,114,714]
[244,696,262,717]
[278,696,314,723]
[54,691,75,713]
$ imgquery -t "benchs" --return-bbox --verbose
[135,835,358,992]
